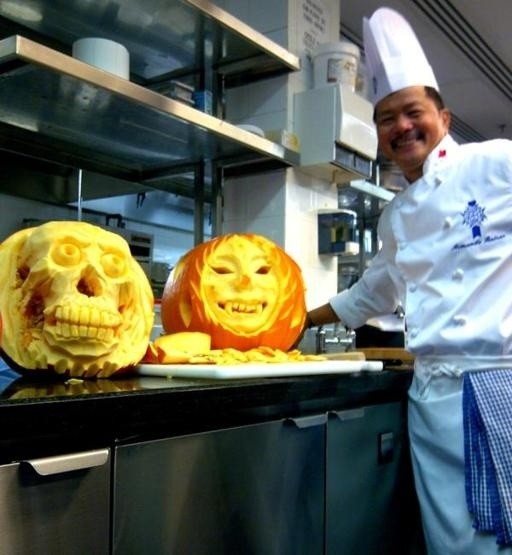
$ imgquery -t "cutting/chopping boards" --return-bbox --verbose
[136,359,384,379]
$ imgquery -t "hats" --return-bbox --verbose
[358,5,441,109]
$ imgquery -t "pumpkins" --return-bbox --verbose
[0,219,155,379]
[160,233,308,352]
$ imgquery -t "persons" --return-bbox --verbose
[307,6,511,554]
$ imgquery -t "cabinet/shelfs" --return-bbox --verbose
[1,0,302,248]
[337,159,396,279]
